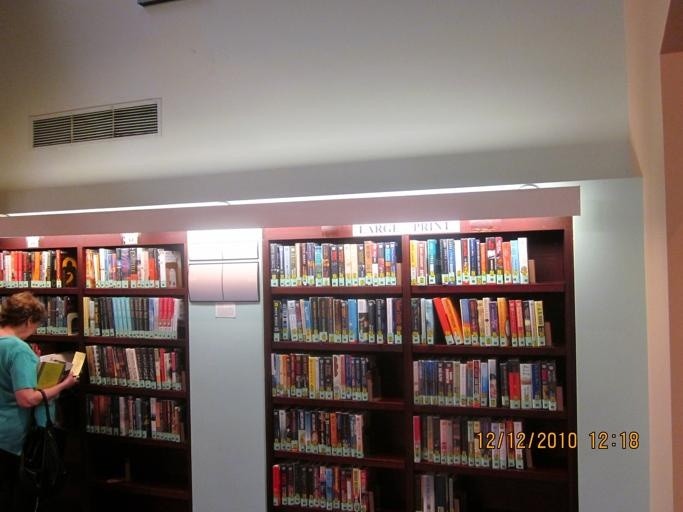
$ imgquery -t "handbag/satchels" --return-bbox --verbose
[19,423,66,495]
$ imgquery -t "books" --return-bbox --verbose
[1,250,81,390]
[270,241,403,512]
[408,237,536,285]
[421,473,460,512]
[85,248,183,444]
[413,415,532,470]
[413,358,564,411]
[410,297,551,347]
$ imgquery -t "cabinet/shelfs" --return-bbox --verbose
[262,216,579,512]
[0,230,192,512]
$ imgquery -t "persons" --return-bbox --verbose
[0,291,81,512]
